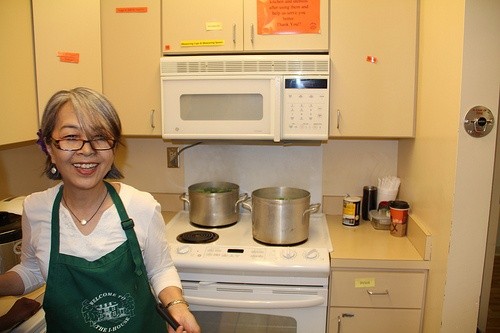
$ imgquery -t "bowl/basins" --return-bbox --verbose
[367,209,392,230]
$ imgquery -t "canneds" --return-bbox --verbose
[342,197,361,226]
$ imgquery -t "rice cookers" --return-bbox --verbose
[0,210,22,275]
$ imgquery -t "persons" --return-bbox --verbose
[0,87,202,333]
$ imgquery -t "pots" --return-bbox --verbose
[180,181,250,228]
[242,187,320,246]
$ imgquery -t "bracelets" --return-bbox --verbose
[165,299,190,311]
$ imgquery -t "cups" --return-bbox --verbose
[375,190,398,210]
[388,200,409,237]
[341,197,362,226]
[362,185,377,220]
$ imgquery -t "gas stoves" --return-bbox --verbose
[163,199,334,254]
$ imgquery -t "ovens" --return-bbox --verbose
[152,249,332,333]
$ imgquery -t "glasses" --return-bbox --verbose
[48,133,118,151]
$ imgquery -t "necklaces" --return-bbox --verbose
[63,187,109,226]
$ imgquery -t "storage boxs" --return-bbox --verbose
[368,209,391,231]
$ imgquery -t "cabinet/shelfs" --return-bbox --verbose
[323,270,428,333]
[328,0,419,141]
[159,0,329,56]
[0,0,103,146]
[100,0,164,138]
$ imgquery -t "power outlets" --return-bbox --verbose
[166,146,180,168]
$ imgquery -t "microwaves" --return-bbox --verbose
[160,53,330,145]
